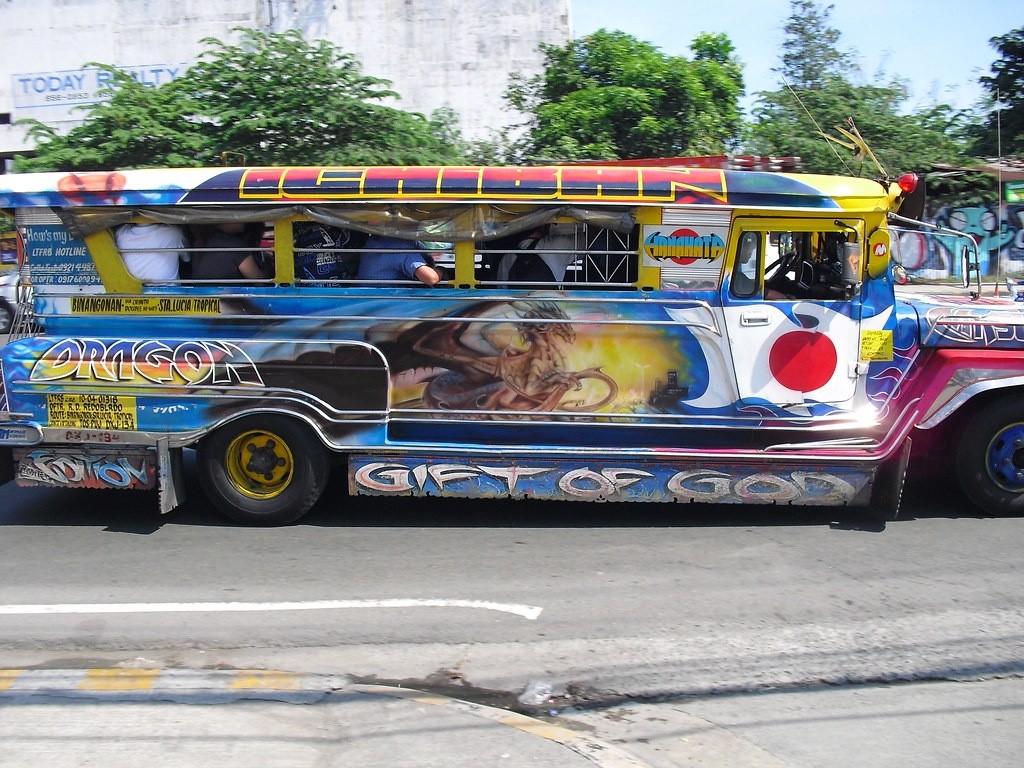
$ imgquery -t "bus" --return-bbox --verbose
[0,145,1024,526]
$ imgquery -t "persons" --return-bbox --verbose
[190,222,269,280]
[734,235,786,299]
[496,225,586,290]
[293,223,354,286]
[114,221,189,285]
[359,232,448,284]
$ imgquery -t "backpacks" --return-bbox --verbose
[508,235,560,290]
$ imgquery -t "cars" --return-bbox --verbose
[0,274,45,333]
